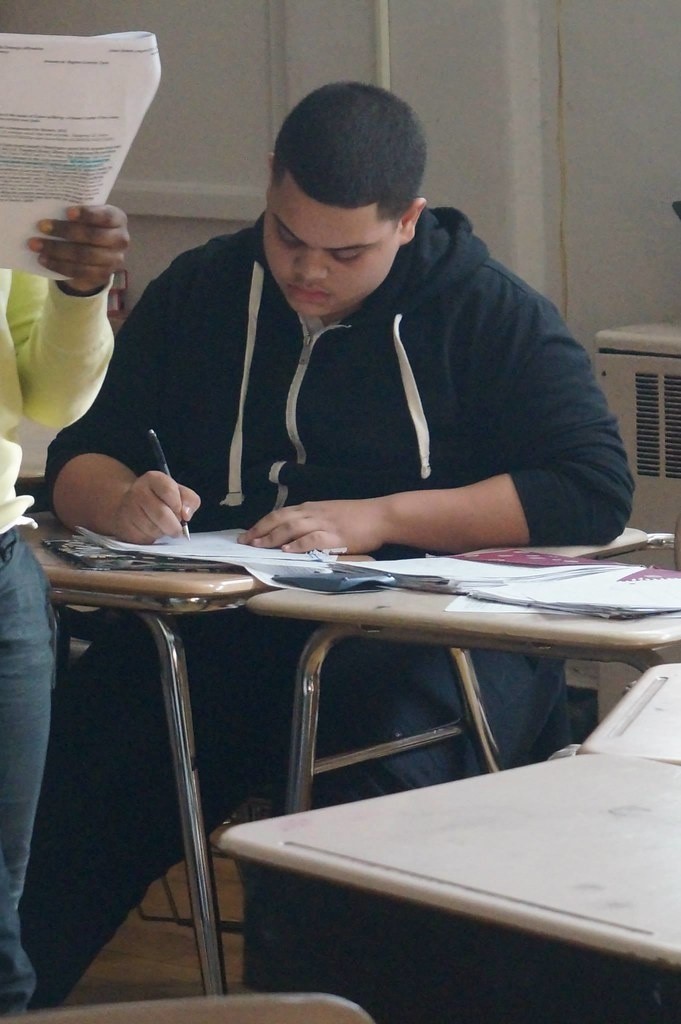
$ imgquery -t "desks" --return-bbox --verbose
[17,509,681,1023]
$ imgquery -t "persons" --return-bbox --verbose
[0,205,128,1013]
[17,81,633,1009]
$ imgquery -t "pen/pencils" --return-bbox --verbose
[146,428,191,542]
